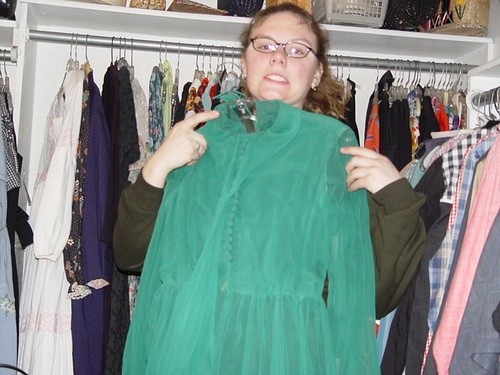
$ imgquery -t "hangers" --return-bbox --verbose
[2,30,500,139]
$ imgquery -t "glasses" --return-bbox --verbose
[247,37,318,58]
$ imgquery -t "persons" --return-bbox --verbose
[112,3,426,375]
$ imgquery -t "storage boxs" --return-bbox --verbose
[418,1,490,37]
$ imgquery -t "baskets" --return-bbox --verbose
[420,0,489,37]
[311,0,388,28]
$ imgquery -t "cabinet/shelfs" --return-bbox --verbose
[0,0,500,303]
